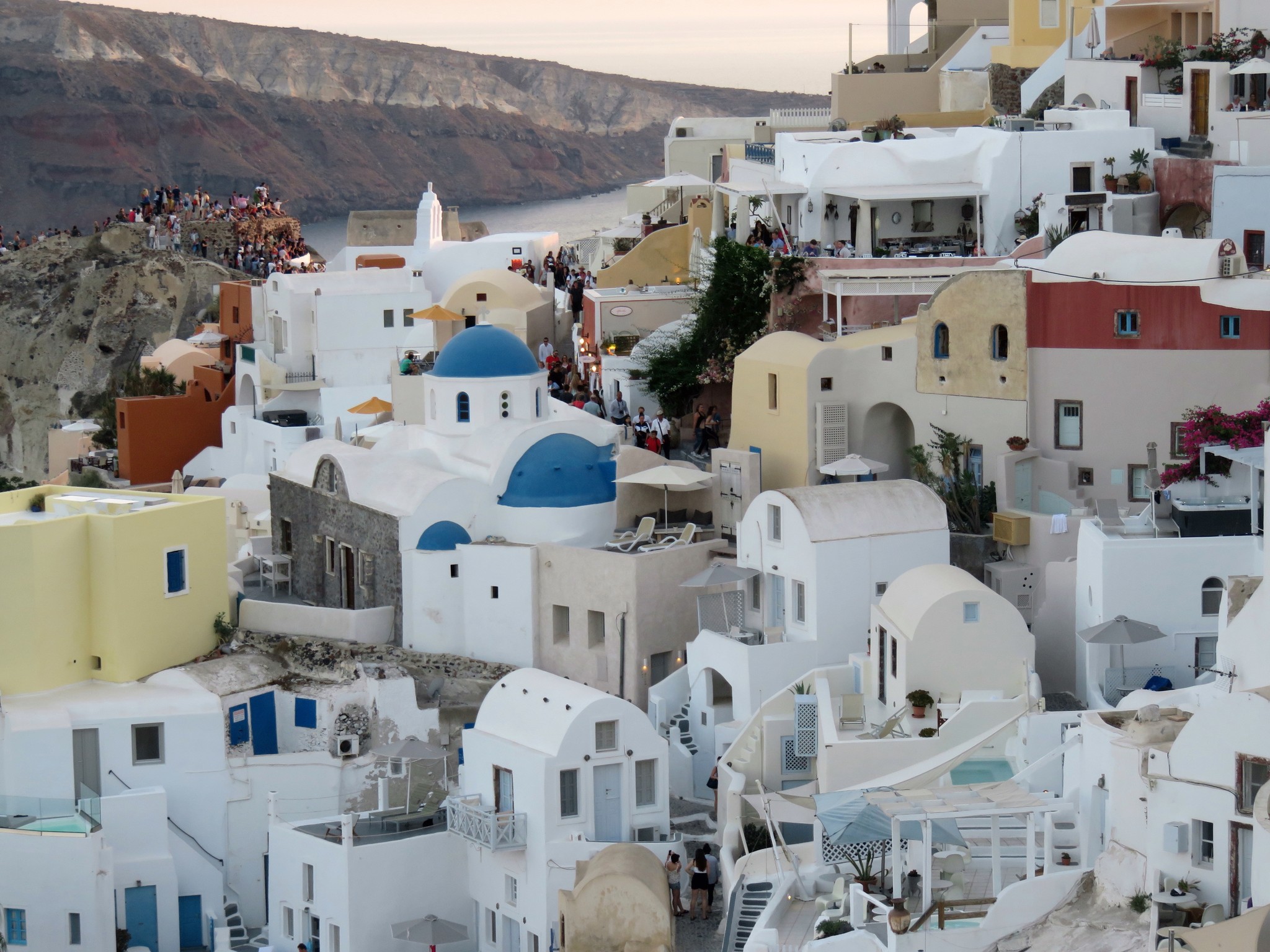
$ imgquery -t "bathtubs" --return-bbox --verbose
[910,910,985,934]
[1171,497,1260,537]
[17,815,94,835]
[949,756,1017,787]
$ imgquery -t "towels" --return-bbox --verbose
[1050,514,1068,534]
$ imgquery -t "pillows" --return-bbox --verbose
[690,509,713,526]
[659,508,688,522]
[633,512,657,527]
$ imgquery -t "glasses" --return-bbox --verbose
[544,341,548,342]
[554,352,558,355]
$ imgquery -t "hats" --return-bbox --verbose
[547,258,553,262]
[577,276,582,280]
[578,265,586,270]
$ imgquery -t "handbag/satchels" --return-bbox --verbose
[707,766,718,789]
[550,382,560,398]
[662,434,670,443]
[691,433,697,443]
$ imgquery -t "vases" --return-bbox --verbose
[888,898,912,935]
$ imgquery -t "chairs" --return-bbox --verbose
[855,717,899,739]
[870,705,910,738]
[368,782,432,829]
[324,811,360,843]
[1117,506,1132,518]
[937,872,964,908]
[458,798,482,833]
[1150,875,1229,952]
[383,788,449,834]
[838,693,866,731]
[637,521,697,553]
[1144,490,1181,538]
[938,854,966,884]
[741,228,979,257]
[956,841,972,866]
[1066,506,1088,516]
[484,810,513,840]
[815,876,845,912]
[404,350,420,366]
[604,516,656,553]
[615,285,656,295]
[1095,499,1127,535]
[820,892,850,919]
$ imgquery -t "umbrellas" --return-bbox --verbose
[809,784,974,900]
[1224,58,1270,96]
[818,453,889,483]
[171,469,185,494]
[188,328,230,369]
[610,464,719,532]
[1084,7,1101,58]
[390,914,469,952]
[405,304,468,364]
[1075,615,1169,691]
[347,397,394,426]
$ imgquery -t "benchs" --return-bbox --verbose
[613,523,716,542]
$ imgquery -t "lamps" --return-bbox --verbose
[677,650,681,662]
[807,197,813,214]
[643,658,648,670]
[824,200,839,220]
[848,201,860,221]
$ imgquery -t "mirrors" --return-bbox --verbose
[912,200,933,233]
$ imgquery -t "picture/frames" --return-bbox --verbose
[787,206,791,225]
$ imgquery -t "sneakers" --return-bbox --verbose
[690,449,711,459]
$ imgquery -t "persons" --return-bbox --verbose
[1244,93,1260,111]
[1078,216,1087,232]
[663,849,689,916]
[0,168,324,282]
[685,849,711,919]
[709,756,720,815]
[735,205,992,266]
[400,353,418,377]
[701,842,720,912]
[519,239,732,465]
[1225,94,1243,112]
[297,943,308,952]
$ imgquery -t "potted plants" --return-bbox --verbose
[1060,852,1071,865]
[906,689,934,718]
[642,212,651,225]
[29,493,45,512]
[876,118,897,141]
[1102,156,1118,194]
[611,238,632,256]
[1006,436,1030,452]
[861,127,877,143]
[1125,147,1151,193]
[671,523,680,531]
[890,115,906,140]
[907,869,921,898]
[843,842,889,894]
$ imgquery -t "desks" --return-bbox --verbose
[1173,495,1260,530]
[1038,121,1071,131]
[730,633,754,643]
[913,880,953,913]
[260,555,292,598]
[405,362,435,376]
[468,805,497,836]
[931,850,966,868]
[653,527,703,543]
[1116,684,1140,696]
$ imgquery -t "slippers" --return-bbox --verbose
[690,916,697,920]
[679,910,689,914]
[703,916,710,920]
[674,912,684,917]
[706,909,712,913]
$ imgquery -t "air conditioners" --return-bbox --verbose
[740,627,760,646]
[632,824,661,841]
[335,734,360,756]
[1223,256,1241,276]
